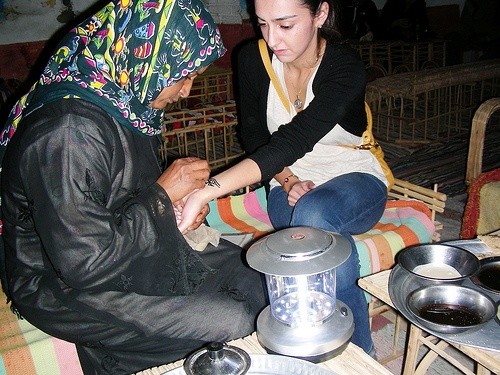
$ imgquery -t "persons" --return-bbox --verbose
[174,0,390,357]
[0,0,271,375]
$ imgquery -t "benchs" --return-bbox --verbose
[18,177,448,375]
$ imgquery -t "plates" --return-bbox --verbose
[469,256,500,295]
[160,354,338,375]
[406,284,497,333]
[398,243,481,287]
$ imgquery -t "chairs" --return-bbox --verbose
[160,39,500,172]
[458,98,500,241]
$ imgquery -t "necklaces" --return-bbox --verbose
[285,58,319,109]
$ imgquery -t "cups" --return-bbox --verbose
[184,346,251,375]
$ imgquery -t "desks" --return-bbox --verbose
[130,331,397,375]
[356,236,500,375]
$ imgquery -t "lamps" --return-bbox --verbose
[246,225,357,365]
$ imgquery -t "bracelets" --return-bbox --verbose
[282,175,298,187]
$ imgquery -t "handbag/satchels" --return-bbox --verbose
[362,101,395,193]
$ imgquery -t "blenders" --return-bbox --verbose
[245,227,354,363]
